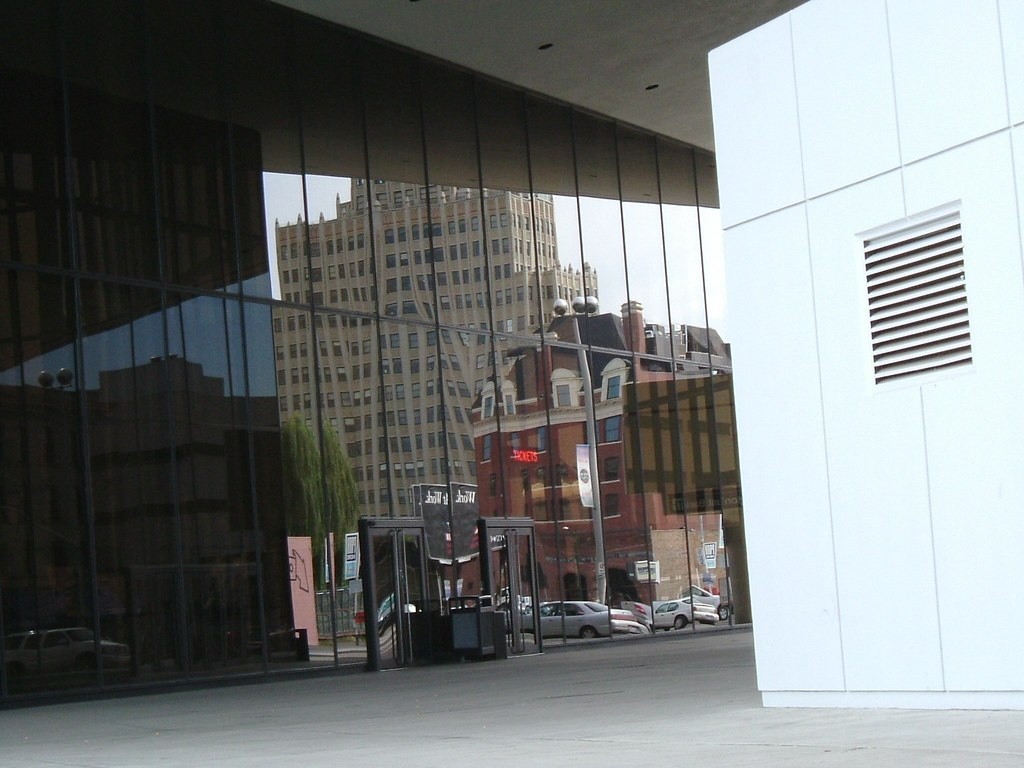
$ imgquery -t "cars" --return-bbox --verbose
[378,593,396,636]
[3,627,134,684]
[477,584,732,637]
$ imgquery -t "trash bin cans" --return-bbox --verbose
[447,594,497,659]
[293,628,312,663]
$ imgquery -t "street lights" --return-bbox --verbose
[562,527,586,603]
[554,296,608,605]
